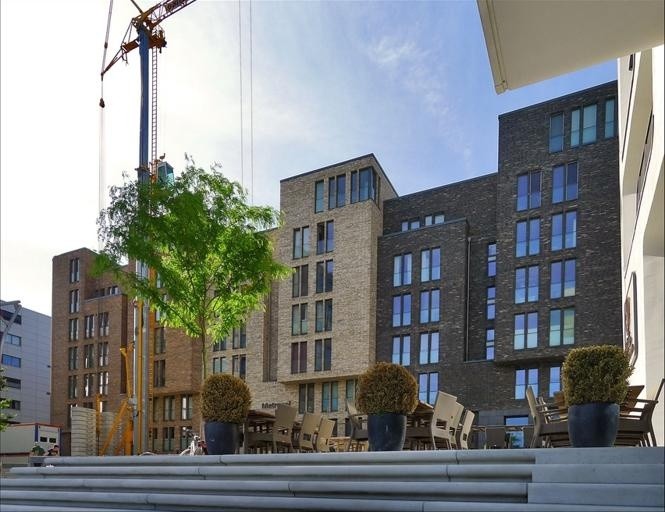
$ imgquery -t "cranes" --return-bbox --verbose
[91,0,201,456]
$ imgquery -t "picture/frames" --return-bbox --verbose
[623,271,638,370]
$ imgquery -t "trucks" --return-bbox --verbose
[1,423,61,468]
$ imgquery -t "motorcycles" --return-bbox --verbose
[138,427,208,459]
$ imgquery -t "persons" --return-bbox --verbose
[29,443,44,467]
[45,444,59,456]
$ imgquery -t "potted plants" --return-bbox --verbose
[559,346,636,447]
[198,373,252,454]
[354,362,419,451]
